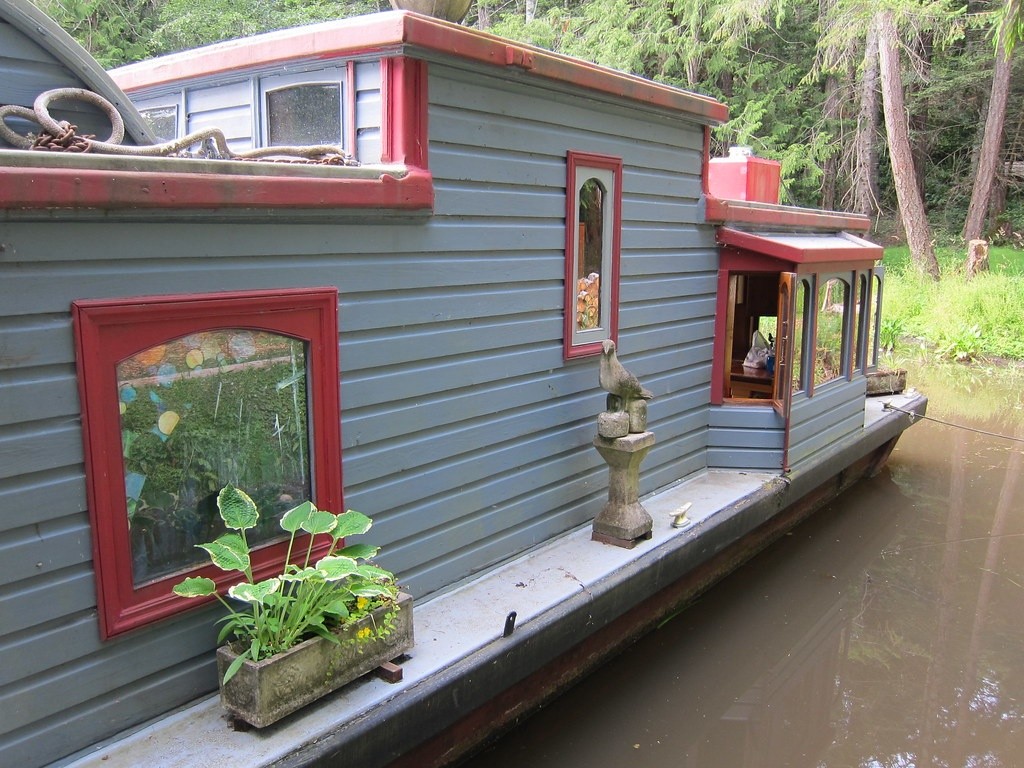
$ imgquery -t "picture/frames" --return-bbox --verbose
[562,146,623,365]
[67,283,350,643]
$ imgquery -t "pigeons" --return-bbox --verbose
[598,339,654,416]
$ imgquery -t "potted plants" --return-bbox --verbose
[174,479,416,734]
